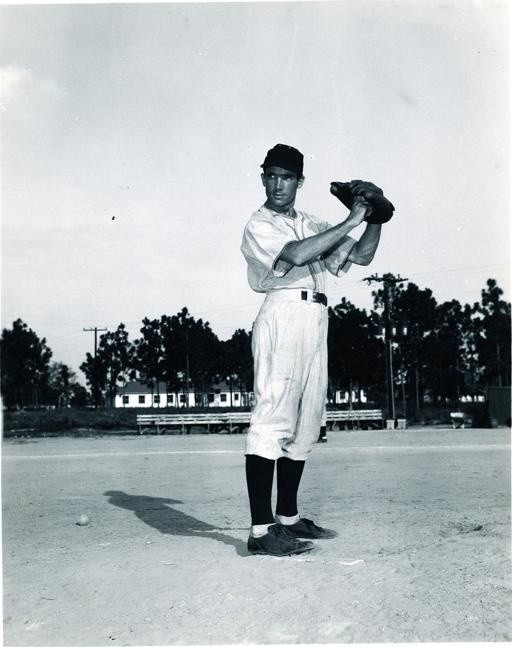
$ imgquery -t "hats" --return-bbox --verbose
[259,141,304,176]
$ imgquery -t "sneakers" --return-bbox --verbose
[275,516,338,540]
[246,522,315,557]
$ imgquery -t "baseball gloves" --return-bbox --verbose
[329,180,395,224]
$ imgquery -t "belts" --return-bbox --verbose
[301,290,327,307]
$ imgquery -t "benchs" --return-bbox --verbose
[136,409,383,436]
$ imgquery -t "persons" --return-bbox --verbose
[318,400,327,442]
[241,140,395,554]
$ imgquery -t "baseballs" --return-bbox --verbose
[77,514,90,526]
